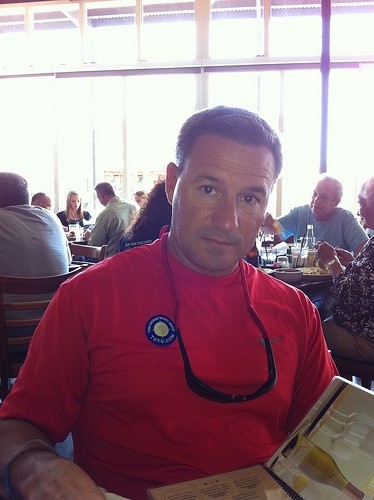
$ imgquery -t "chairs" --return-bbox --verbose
[0,243,107,404]
[330,353,374,390]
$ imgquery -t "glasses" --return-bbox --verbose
[176,305,277,404]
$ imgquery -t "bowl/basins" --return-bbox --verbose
[274,268,303,283]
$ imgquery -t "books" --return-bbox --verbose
[147,376,374,500]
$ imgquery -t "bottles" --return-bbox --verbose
[282,433,364,500]
[304,224,316,251]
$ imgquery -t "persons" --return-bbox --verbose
[0,105,374,500]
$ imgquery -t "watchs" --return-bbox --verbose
[326,259,340,270]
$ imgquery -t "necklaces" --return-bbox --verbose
[71,213,77,218]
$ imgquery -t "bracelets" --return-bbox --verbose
[3,439,59,499]
